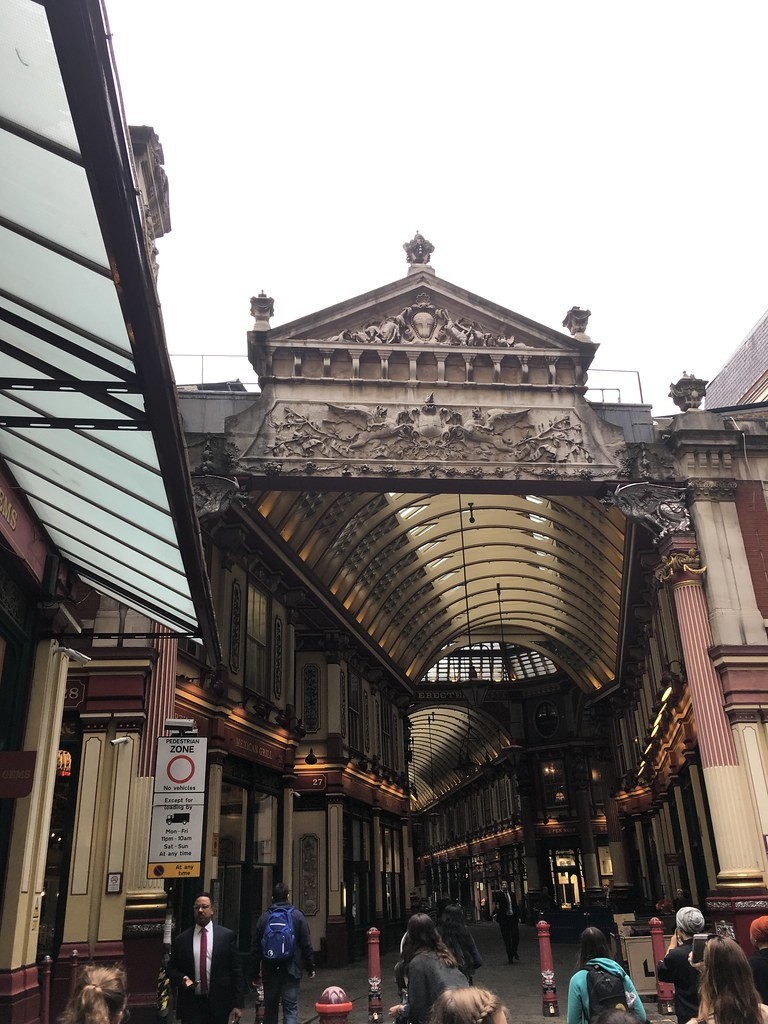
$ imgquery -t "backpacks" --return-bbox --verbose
[576,965,632,1024]
[261,906,295,961]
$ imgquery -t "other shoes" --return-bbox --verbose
[514,952,519,960]
[509,959,513,963]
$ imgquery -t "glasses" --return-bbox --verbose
[193,904,212,912]
[706,934,740,946]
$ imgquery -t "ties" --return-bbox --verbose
[505,891,510,914]
[200,928,207,999]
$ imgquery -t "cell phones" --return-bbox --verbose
[690,934,708,967]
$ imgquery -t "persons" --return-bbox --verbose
[167,892,246,1024]
[388,877,768,1024]
[247,882,315,1024]
[58,964,129,1024]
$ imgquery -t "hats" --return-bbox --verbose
[676,907,705,932]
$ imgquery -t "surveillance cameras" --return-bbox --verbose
[66,648,92,665]
[293,792,301,798]
[109,737,129,746]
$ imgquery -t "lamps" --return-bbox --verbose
[458,493,477,679]
[179,673,317,765]
[608,660,684,799]
[425,716,444,826]
[348,752,419,802]
[452,707,495,771]
